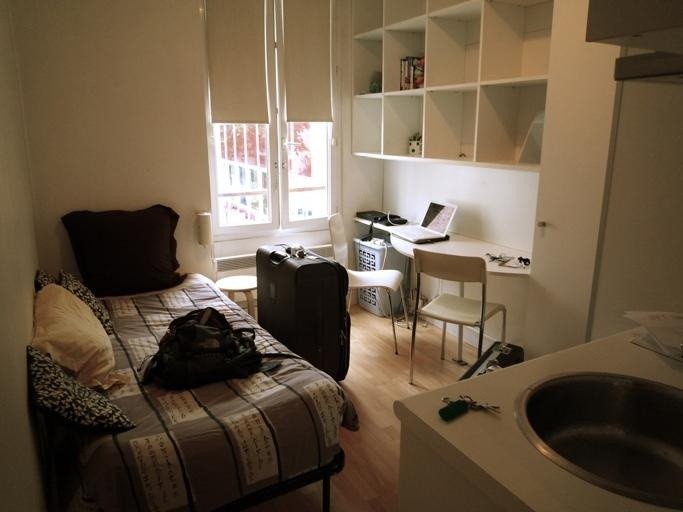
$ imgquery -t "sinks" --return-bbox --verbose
[512,372,683,510]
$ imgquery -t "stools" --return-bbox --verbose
[216,275,257,319]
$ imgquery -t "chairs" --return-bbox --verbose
[408,248,506,384]
[327,212,410,355]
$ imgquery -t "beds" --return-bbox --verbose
[49,271,359,512]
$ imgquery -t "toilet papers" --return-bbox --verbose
[195,210,213,247]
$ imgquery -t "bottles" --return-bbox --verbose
[398,58,411,91]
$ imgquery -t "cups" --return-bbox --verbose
[408,140,421,155]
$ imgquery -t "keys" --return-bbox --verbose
[438,394,501,422]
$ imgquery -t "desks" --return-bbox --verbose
[352,213,532,354]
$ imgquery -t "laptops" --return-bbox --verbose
[390,200,458,243]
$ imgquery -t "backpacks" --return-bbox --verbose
[141,306,261,388]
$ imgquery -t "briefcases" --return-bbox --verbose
[455,340,524,388]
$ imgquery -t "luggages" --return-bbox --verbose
[254,242,349,384]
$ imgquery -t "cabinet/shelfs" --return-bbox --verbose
[349,0,554,172]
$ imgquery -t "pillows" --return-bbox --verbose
[60,267,113,335]
[32,283,130,391]
[35,268,58,288]
[27,344,137,429]
[61,204,187,297]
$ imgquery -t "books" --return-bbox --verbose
[399,56,425,90]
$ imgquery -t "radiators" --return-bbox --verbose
[215,243,336,310]
